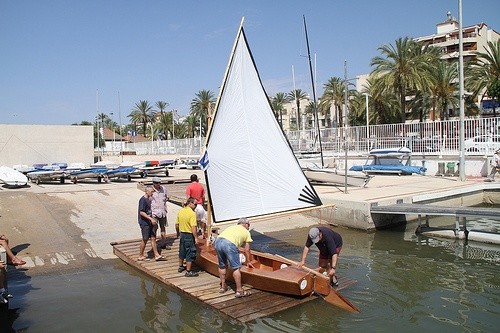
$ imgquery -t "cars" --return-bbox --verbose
[462,134,500,155]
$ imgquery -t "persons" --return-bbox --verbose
[183,202,213,238]
[185,174,205,206]
[175,197,201,277]
[0,232,27,303]
[214,217,255,297]
[297,226,343,286]
[137,187,168,261]
[150,178,170,248]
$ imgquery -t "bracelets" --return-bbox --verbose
[331,266,336,270]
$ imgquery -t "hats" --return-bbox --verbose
[152,178,161,183]
[309,228,320,243]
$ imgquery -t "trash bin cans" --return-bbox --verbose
[458,163,465,176]
[437,162,445,175]
[447,161,455,176]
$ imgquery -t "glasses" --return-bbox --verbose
[192,202,197,206]
[246,223,250,227]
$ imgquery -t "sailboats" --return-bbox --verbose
[188,17,361,314]
[291,53,375,187]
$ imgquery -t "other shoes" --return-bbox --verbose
[185,271,199,277]
[178,266,186,272]
[0,288,13,303]
[7,257,26,266]
[166,244,171,250]
[219,286,228,294]
[235,288,252,297]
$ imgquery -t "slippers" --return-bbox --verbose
[138,257,151,261]
[155,256,167,261]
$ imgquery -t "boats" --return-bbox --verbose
[0,165,28,187]
[21,158,204,185]
[350,147,428,175]
